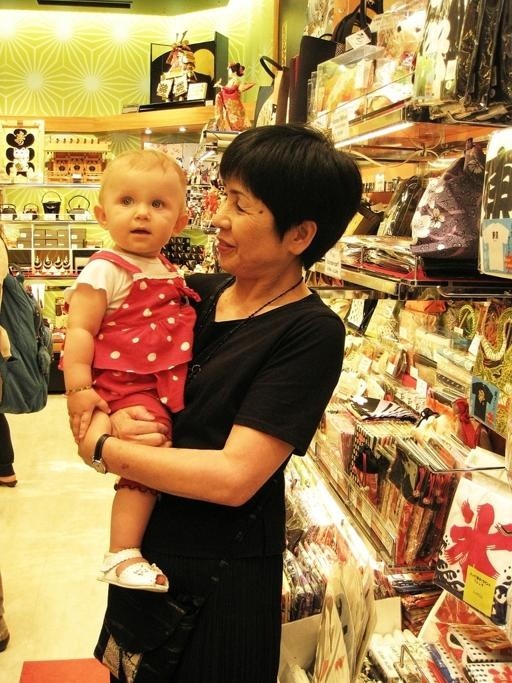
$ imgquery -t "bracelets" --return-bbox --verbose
[64,384,91,396]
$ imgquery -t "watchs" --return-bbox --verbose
[91,433,113,474]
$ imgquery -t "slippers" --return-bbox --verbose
[1,479,18,488]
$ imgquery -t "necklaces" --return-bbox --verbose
[186,275,302,385]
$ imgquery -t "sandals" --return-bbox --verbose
[98,547,171,595]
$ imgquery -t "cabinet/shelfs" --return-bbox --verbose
[253,90,508,683]
[3,106,251,395]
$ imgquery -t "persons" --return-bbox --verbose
[62,149,204,592]
[0,228,20,490]
[211,62,247,132]
[89,119,366,683]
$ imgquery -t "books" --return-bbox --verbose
[283,394,512,683]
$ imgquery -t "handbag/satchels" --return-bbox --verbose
[1,272,51,414]
[410,136,485,260]
